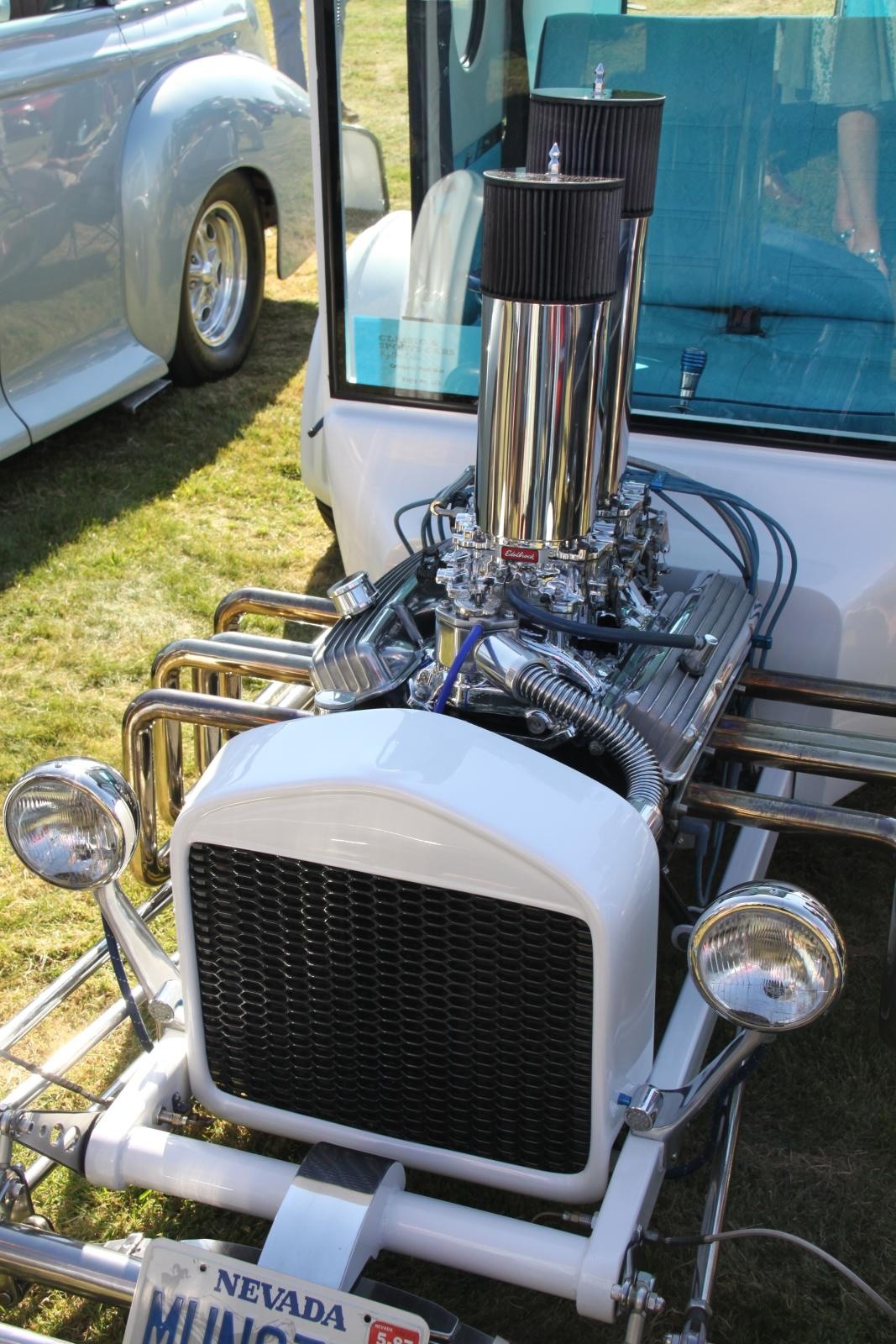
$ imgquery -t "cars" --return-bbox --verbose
[0,0,895,1343]
[0,0,389,460]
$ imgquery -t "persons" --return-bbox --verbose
[831,0,896,278]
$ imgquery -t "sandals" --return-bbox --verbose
[857,248,889,283]
[760,162,799,206]
[829,218,857,252]
